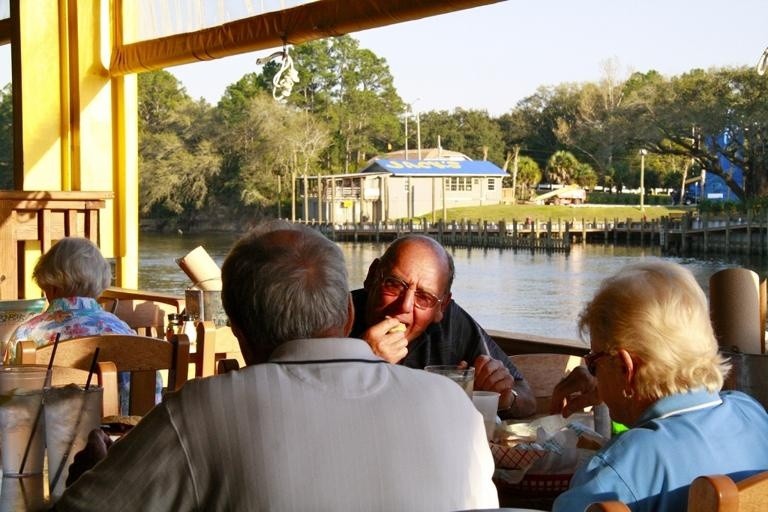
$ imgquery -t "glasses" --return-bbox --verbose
[382,262,445,309]
[583,351,604,375]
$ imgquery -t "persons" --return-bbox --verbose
[350,235,537,418]
[7,237,162,417]
[52,221,501,510]
[550,260,768,512]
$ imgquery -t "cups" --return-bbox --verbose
[471,391,500,441]
[44,383,105,501]
[0,363,53,477]
[424,364,475,399]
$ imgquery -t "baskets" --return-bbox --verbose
[493,474,571,498]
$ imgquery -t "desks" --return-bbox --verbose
[0,190,115,304]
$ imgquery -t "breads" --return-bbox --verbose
[386,322,407,334]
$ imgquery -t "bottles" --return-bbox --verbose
[180,315,198,355]
[166,313,181,337]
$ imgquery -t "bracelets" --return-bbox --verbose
[497,389,518,414]
